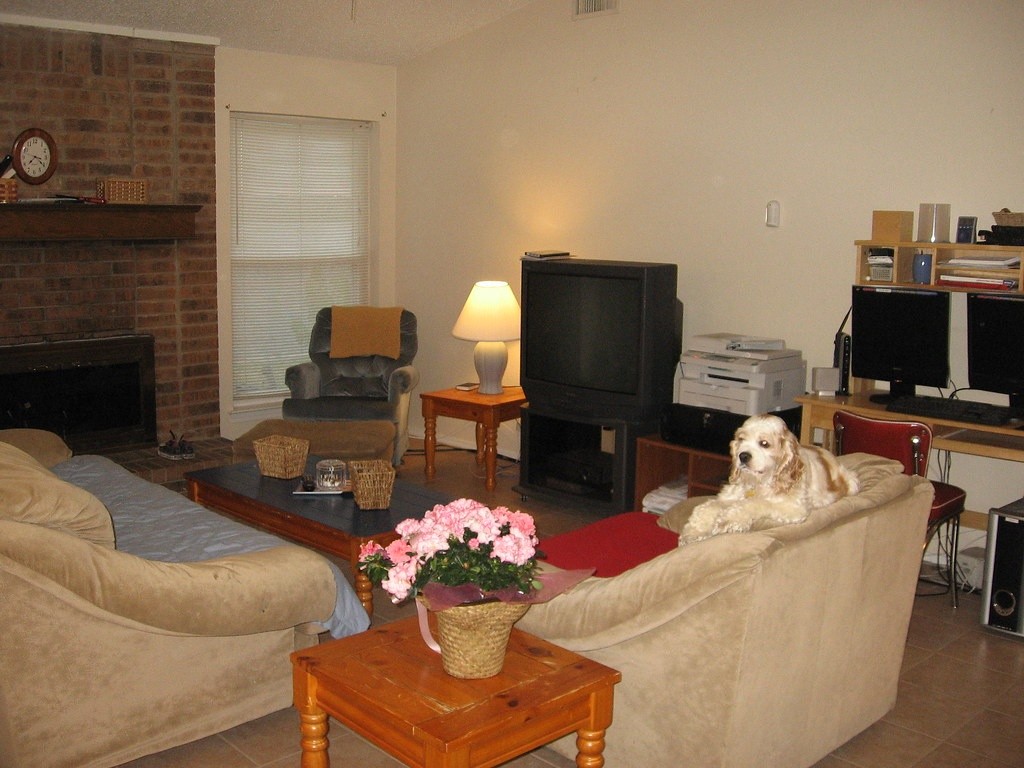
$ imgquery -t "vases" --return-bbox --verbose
[913,254,930,282]
[411,585,537,680]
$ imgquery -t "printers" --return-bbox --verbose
[673,333,807,416]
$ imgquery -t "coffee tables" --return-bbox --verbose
[183,452,447,621]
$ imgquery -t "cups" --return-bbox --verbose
[913,254,932,284]
[316,459,346,492]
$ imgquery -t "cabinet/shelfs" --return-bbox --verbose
[847,239,1024,396]
[632,439,731,517]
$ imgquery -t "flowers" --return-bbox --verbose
[355,496,551,610]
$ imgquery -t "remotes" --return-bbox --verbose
[302,475,315,492]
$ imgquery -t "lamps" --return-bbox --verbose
[452,280,522,396]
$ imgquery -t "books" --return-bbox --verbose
[456,383,480,391]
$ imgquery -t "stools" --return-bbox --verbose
[231,414,400,468]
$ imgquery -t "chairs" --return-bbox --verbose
[828,408,967,610]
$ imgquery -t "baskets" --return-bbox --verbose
[416,593,530,679]
[992,208,1024,226]
[253,434,311,479]
[97,175,148,205]
[348,459,397,509]
[0,177,17,201]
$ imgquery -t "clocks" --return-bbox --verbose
[11,126,61,184]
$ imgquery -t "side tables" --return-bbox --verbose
[285,613,622,768]
[418,383,529,492]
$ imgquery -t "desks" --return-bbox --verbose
[793,388,1024,463]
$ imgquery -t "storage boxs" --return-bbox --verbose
[871,210,914,241]
[869,266,892,281]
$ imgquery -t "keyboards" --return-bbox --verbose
[886,393,1017,427]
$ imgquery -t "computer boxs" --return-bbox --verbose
[979,495,1024,638]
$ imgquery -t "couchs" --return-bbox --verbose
[512,449,938,768]
[282,303,421,470]
[0,426,369,768]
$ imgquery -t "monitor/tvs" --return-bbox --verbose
[966,292,1024,421]
[851,284,950,404]
[520,257,683,422]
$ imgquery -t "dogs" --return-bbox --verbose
[686,412,859,535]
